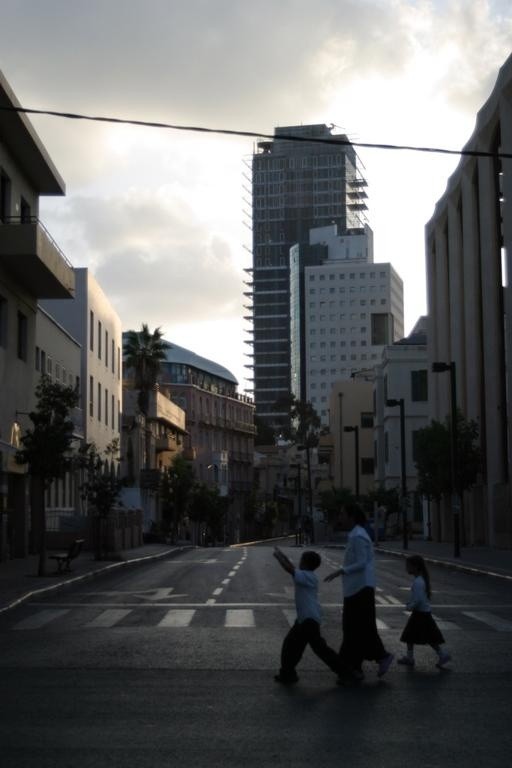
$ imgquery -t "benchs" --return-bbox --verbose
[48,539,87,572]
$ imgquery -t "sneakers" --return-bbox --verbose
[396,657,415,664]
[335,671,365,685]
[273,674,298,683]
[435,655,451,666]
[376,653,393,676]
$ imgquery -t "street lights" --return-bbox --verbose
[289,445,315,545]
[385,398,408,549]
[343,425,359,495]
[207,464,218,547]
[433,361,461,559]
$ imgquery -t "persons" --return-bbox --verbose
[394,554,452,669]
[268,545,343,684]
[318,497,393,678]
